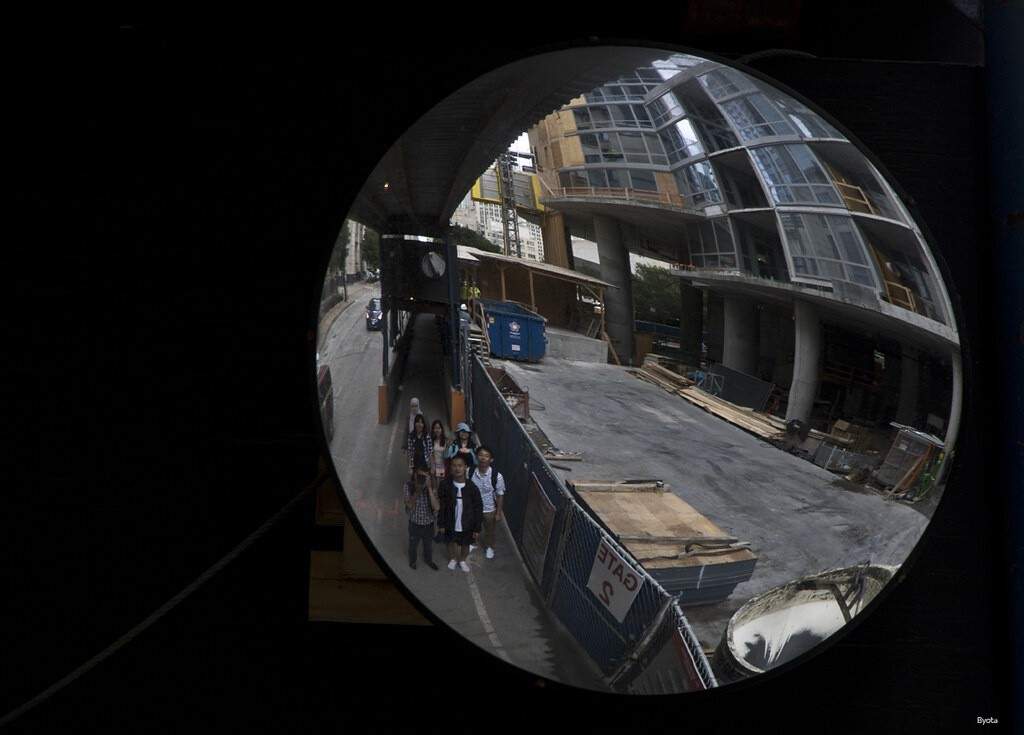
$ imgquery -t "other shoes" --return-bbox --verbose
[410,562,417,570]
[430,564,439,571]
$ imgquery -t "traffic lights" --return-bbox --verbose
[345,225,351,256]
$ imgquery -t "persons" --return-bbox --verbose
[407,414,434,479]
[437,456,483,571]
[430,420,449,545]
[466,447,506,558]
[443,423,478,465]
[401,398,429,454]
[403,466,439,571]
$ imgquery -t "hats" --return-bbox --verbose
[454,423,471,432]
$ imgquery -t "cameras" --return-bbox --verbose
[418,475,426,483]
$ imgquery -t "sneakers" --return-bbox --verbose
[459,562,469,571]
[486,547,494,558]
[448,560,457,569]
[469,545,477,551]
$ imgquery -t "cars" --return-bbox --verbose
[316,353,333,445]
[363,269,380,282]
[365,298,382,331]
